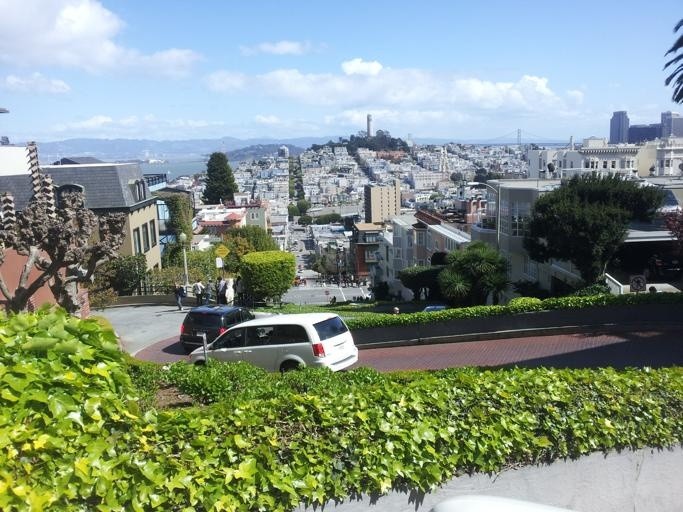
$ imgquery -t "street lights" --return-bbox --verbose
[466,180,500,252]
[179,232,191,286]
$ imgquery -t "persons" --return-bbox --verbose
[216,275,244,306]
[194,279,205,306]
[393,306,400,314]
[175,284,185,311]
[204,280,215,304]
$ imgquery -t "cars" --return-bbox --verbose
[180,305,256,353]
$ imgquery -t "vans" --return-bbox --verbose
[189,311,359,373]
